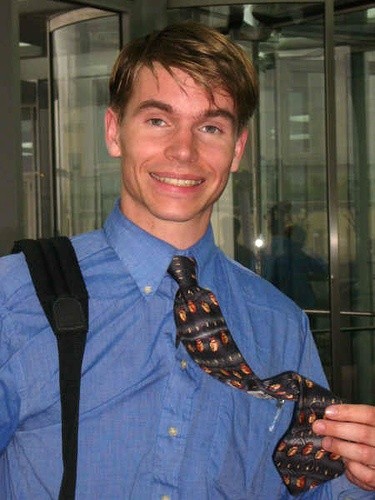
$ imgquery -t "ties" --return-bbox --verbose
[168,254,345,494]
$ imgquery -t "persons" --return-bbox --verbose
[267,225,328,344]
[1,22,375,500]
[217,217,257,274]
